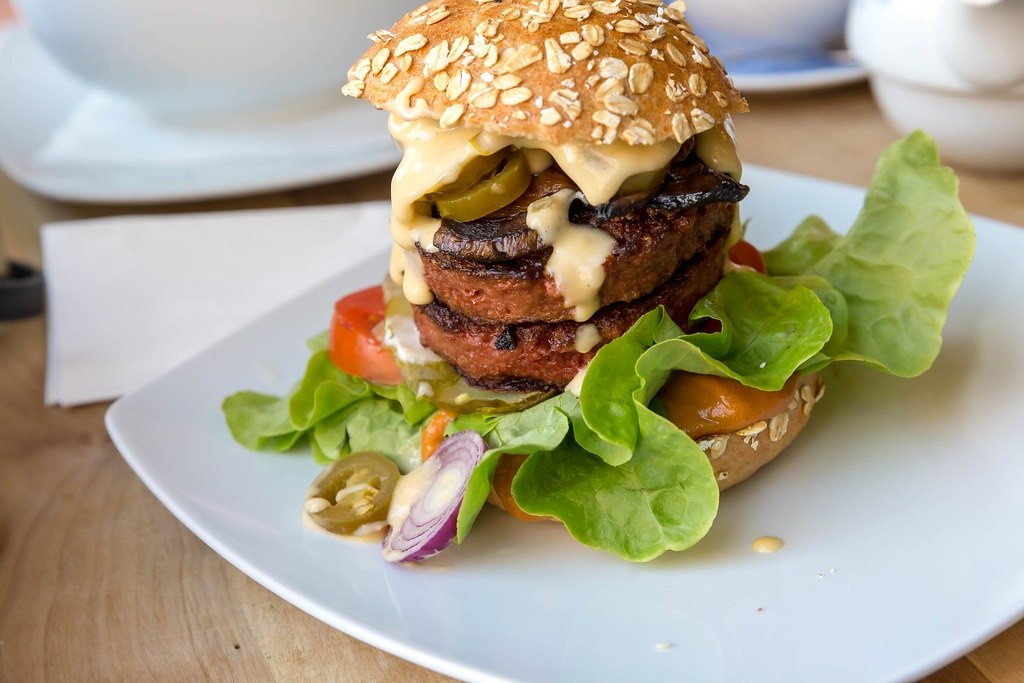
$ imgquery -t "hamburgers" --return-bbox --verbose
[218,0,980,562]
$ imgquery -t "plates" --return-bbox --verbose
[664,46,865,94]
[0,21,458,204]
[103,154,1024,683]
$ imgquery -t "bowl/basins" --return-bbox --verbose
[687,0,839,55]
[8,0,424,126]
[844,0,1024,175]
